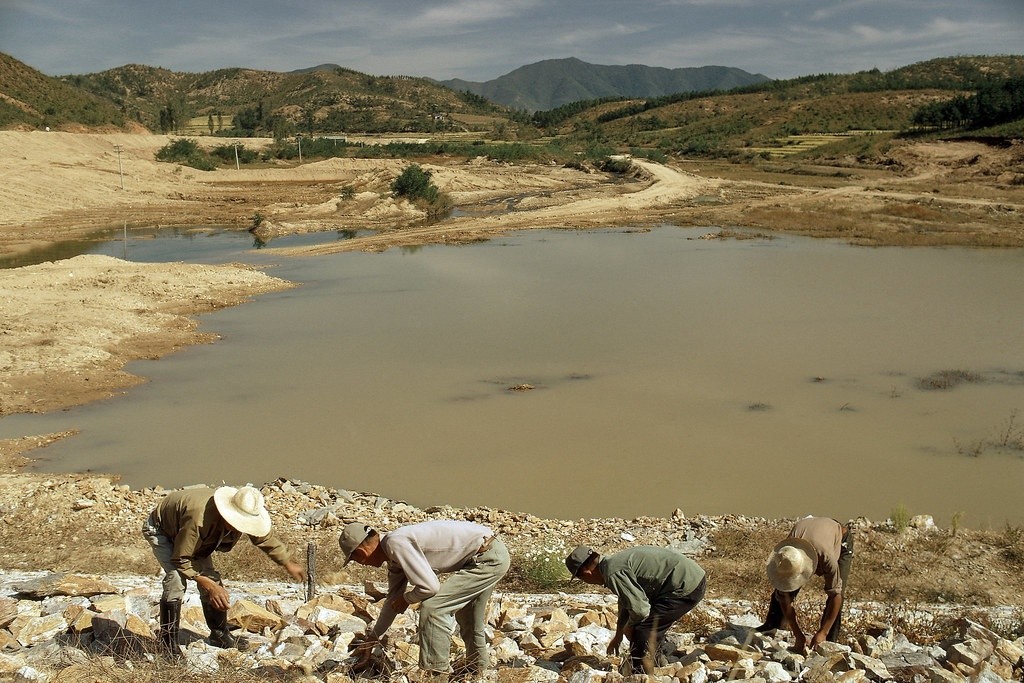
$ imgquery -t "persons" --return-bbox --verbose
[338,520,510,681]
[142,486,308,666]
[566,545,706,674]
[753,515,853,654]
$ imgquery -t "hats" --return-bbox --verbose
[214,486,271,538]
[566,545,593,583]
[339,522,372,567]
[765,537,818,592]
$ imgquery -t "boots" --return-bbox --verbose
[160,599,183,656]
[202,600,250,650]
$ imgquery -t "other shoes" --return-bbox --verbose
[754,624,777,637]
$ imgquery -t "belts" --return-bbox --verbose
[153,509,169,537]
[476,534,498,554]
